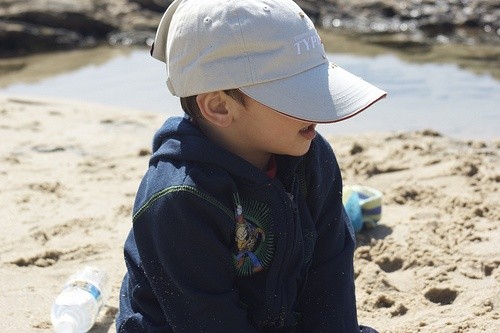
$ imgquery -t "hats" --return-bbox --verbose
[150,0,387,124]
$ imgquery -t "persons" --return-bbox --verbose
[112,0,390,333]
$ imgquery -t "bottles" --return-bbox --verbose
[52,268,106,333]
